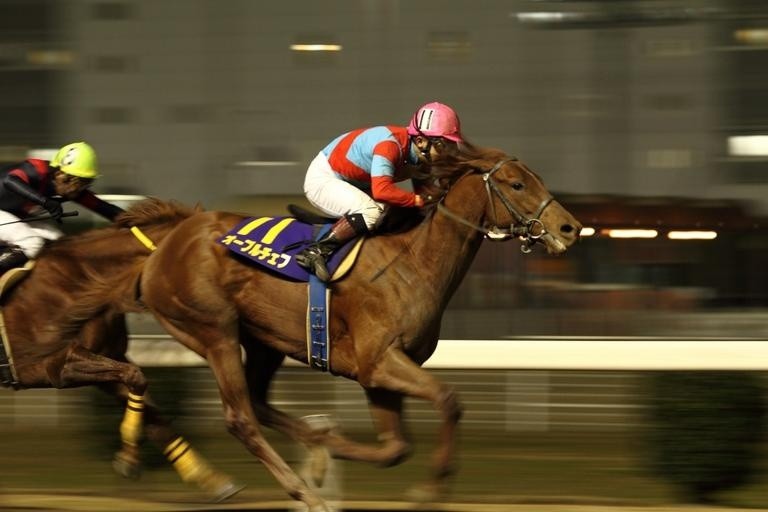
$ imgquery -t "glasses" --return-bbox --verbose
[415,113,444,153]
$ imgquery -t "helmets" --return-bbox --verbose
[408,102,464,142]
[48,141,100,179]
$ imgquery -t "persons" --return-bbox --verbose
[295,101,464,282]
[0,141,127,271]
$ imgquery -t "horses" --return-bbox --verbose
[60,137,582,511]
[0,200,251,503]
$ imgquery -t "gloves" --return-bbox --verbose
[415,191,446,207]
[44,198,63,223]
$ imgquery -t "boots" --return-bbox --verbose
[295,214,368,282]
